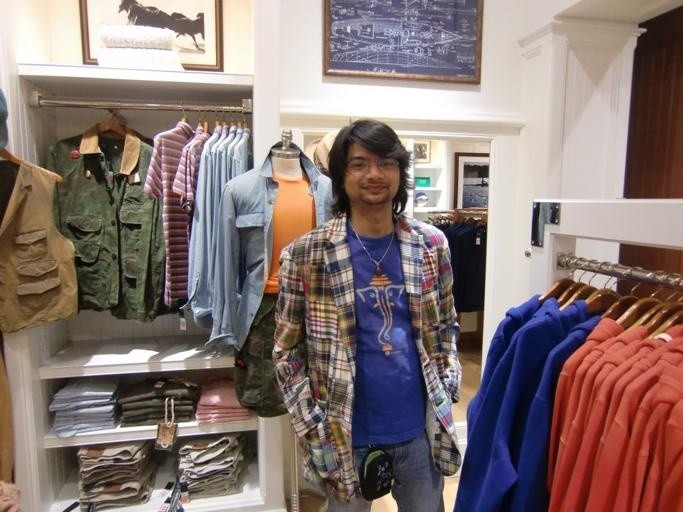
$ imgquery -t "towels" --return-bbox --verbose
[75,436,151,510]
[177,433,246,501]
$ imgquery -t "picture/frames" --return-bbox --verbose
[321,1,483,86]
[79,0,222,71]
[416,139,430,164]
[453,152,489,211]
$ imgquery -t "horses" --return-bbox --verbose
[118,0,204,51]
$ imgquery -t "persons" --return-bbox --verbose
[271,120,463,512]
[203,146,341,511]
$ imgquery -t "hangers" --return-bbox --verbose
[94,104,249,136]
[0,146,64,182]
[499,251,682,342]
[419,210,487,231]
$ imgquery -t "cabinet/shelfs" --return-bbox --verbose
[414,162,444,212]
[41,335,267,509]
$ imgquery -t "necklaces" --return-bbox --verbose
[350,221,396,275]
